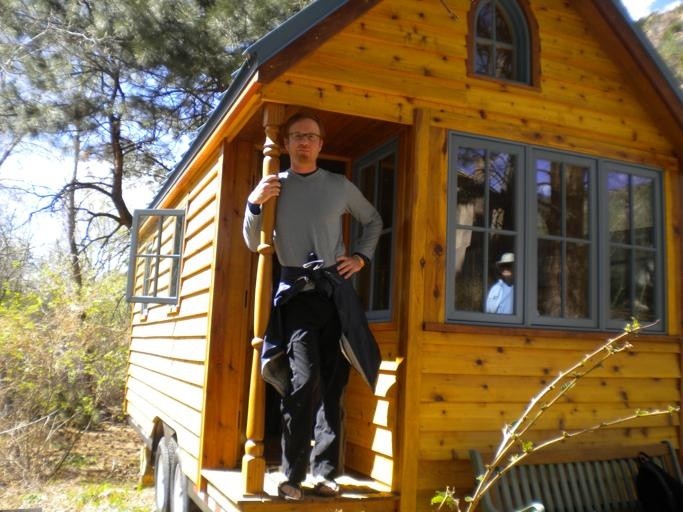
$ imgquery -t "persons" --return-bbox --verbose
[242,113,384,502]
[485,252,515,315]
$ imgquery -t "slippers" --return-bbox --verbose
[278,478,304,504]
[314,480,340,496]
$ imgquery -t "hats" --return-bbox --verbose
[496,253,515,270]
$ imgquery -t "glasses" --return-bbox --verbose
[287,132,320,142]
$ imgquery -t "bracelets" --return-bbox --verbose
[354,255,364,268]
